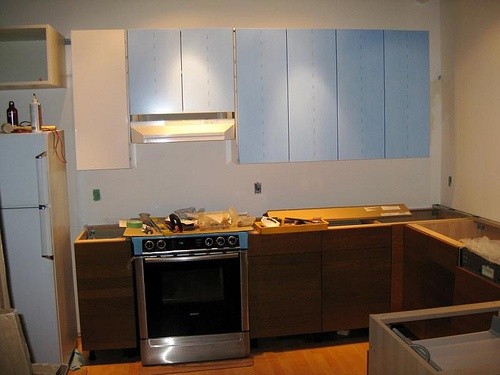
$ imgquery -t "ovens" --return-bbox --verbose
[134,249,250,366]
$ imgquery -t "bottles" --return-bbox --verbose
[7,100,18,127]
[29,92,42,132]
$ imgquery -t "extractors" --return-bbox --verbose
[131,114,236,144]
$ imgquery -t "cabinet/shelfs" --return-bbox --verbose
[70,29,136,171]
[403,218,500,311]
[337,29,431,161]
[127,28,235,116]
[235,28,338,166]
[74,223,137,361]
[366,300,500,375]
[249,217,392,349]
[0,23,67,91]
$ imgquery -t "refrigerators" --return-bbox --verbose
[0,130,78,366]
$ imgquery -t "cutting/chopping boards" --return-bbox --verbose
[123,226,254,237]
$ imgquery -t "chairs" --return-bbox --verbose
[0,309,61,375]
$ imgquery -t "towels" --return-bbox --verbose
[69,348,86,371]
